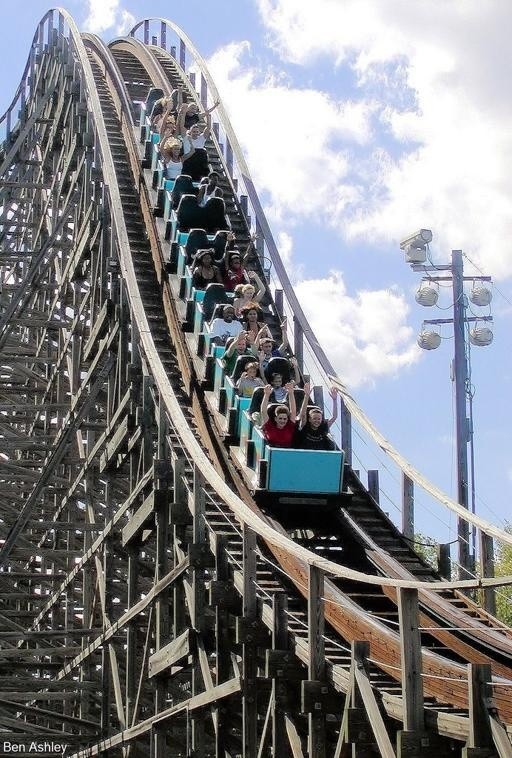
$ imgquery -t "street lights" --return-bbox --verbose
[418,276,496,600]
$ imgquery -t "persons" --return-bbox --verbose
[193,231,338,449]
[153,84,223,205]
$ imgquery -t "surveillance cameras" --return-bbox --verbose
[399,228,433,251]
